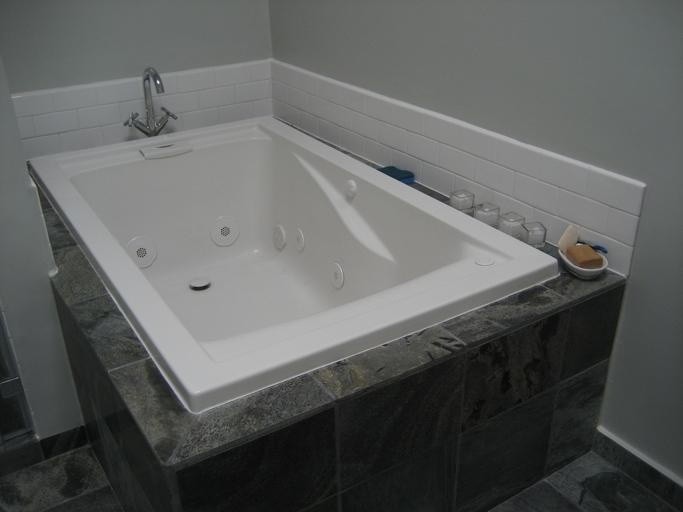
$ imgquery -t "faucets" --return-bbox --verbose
[122,67,178,138]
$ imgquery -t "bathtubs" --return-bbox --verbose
[27,109,562,418]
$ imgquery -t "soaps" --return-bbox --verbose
[567,244,602,268]
